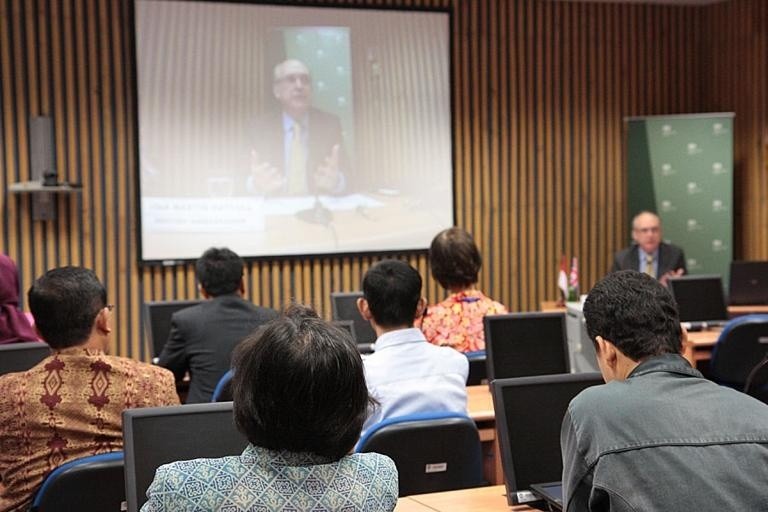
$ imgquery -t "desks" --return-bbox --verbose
[464,383,506,486]
[568,300,601,373]
[394,484,544,512]
[681,307,767,368]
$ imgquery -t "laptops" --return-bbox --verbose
[730,262,768,305]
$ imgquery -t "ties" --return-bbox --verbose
[645,256,656,278]
[288,121,304,182]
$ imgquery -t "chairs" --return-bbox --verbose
[353,411,486,493]
[213,369,236,404]
[708,315,766,402]
[463,350,489,385]
[27,451,127,511]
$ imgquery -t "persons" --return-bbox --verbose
[610,210,688,287]
[350,259,469,454]
[0,265,183,512]
[139,304,400,512]
[154,247,279,405]
[559,269,768,511]
[414,227,510,358]
[233,58,362,197]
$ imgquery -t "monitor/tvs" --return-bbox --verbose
[0,342,54,376]
[121,402,248,512]
[665,276,729,328]
[483,312,571,391]
[143,300,207,365]
[490,372,607,507]
[330,293,378,345]
